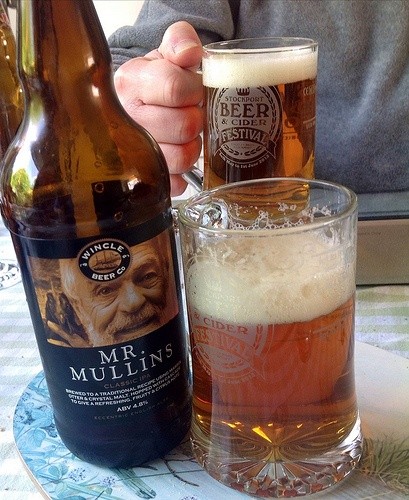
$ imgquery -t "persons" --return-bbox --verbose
[61,234,172,347]
[102,0,409,202]
[42,276,78,336]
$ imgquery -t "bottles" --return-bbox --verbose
[0,0,192,468]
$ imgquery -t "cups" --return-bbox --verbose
[172,34,320,228]
[175,177,363,498]
